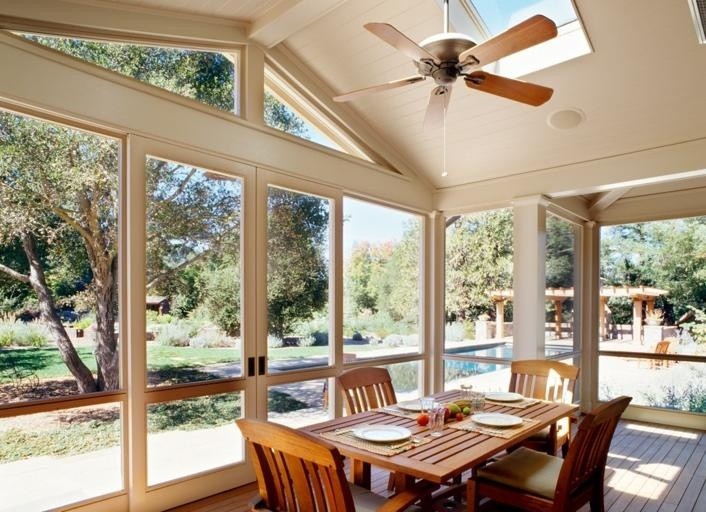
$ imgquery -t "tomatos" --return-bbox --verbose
[456,413,463,421]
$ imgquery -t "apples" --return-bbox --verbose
[417,414,429,425]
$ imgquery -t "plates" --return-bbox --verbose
[485,392,523,401]
[398,401,441,411]
[470,413,523,427]
[353,425,411,442]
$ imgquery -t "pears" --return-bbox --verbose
[448,404,461,415]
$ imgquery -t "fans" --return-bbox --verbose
[331,0,557,142]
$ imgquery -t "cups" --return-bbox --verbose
[428,408,446,438]
[460,383,473,400]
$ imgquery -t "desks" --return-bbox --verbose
[297,387,579,508]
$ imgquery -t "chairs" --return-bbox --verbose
[463,396,631,512]
[237,415,443,512]
[337,366,400,484]
[637,340,670,369]
[506,360,582,456]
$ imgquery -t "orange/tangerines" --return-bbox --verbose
[463,406,471,415]
